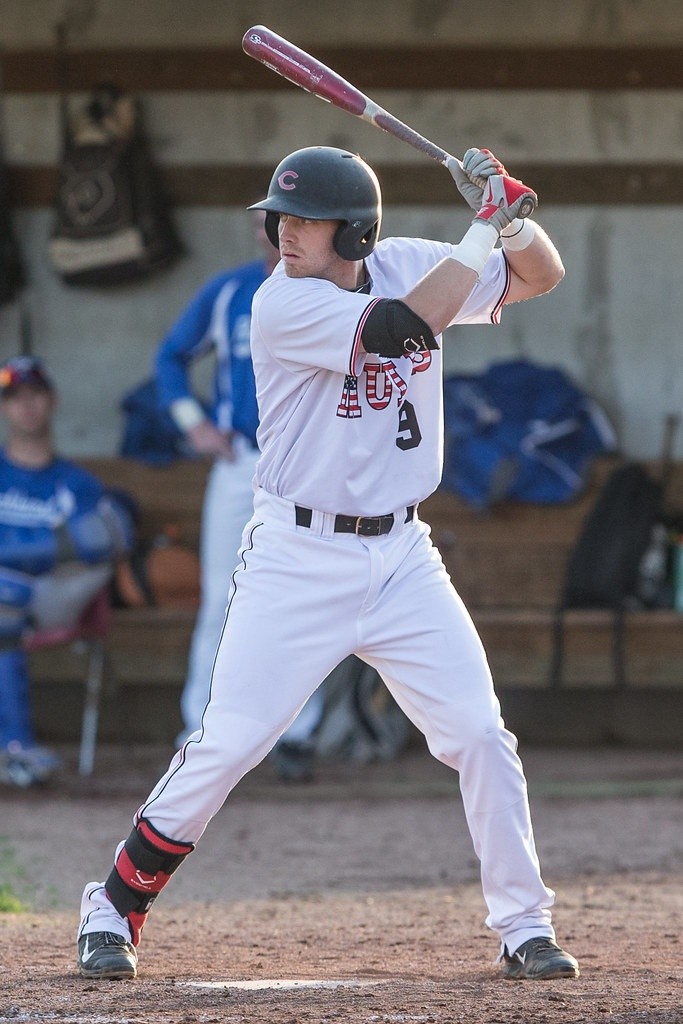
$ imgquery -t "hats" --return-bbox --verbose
[0,356,51,397]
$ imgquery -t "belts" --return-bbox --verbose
[295,506,415,536]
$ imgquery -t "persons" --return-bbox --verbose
[75,146,580,979]
[0,358,133,787]
[153,207,327,782]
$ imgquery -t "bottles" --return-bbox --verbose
[144,524,201,608]
[637,525,683,616]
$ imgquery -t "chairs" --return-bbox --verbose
[24,592,115,778]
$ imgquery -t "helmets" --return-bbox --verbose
[246,146,382,261]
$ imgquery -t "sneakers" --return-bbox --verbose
[78,930,135,979]
[503,932,579,978]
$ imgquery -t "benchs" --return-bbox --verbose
[78,456,682,627]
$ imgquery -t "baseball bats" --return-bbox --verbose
[78,648,107,777]
[239,23,536,221]
[660,414,678,498]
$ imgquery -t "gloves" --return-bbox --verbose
[472,174,538,237]
[448,148,510,211]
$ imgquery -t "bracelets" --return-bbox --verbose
[499,218,535,251]
[450,221,500,278]
[172,399,207,433]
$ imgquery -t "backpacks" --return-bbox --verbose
[557,457,672,620]
[48,78,189,290]
